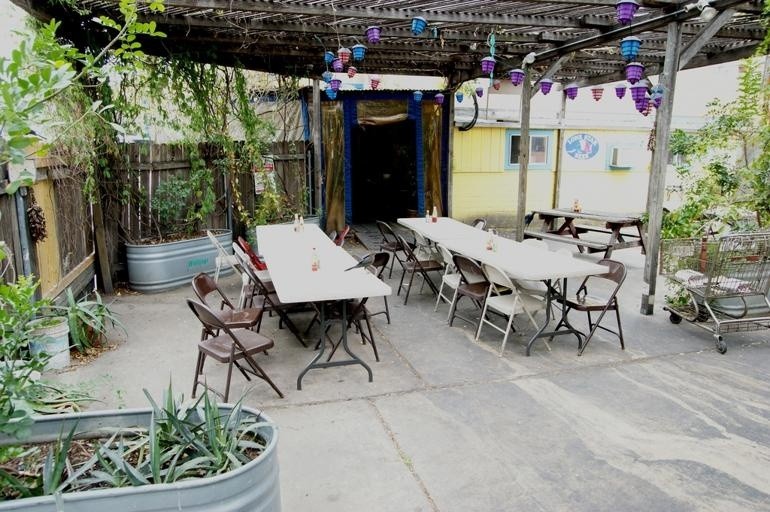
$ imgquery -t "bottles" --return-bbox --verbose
[425,209,431,222]
[310,246,319,272]
[432,206,438,223]
[293,213,304,233]
[485,227,498,252]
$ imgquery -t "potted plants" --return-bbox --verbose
[119,172,239,297]
[42,269,130,357]
[0,272,284,511]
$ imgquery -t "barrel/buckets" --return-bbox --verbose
[25,317,70,368]
[0,360,35,419]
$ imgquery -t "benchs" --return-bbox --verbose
[524,208,647,259]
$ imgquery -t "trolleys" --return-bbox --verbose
[659,232,770,354]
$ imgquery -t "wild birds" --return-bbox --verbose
[344,252,377,271]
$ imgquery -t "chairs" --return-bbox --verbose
[476,262,551,357]
[549,259,626,356]
[397,215,610,349]
[205,223,392,390]
[377,219,418,279]
[185,299,284,403]
[191,273,267,375]
